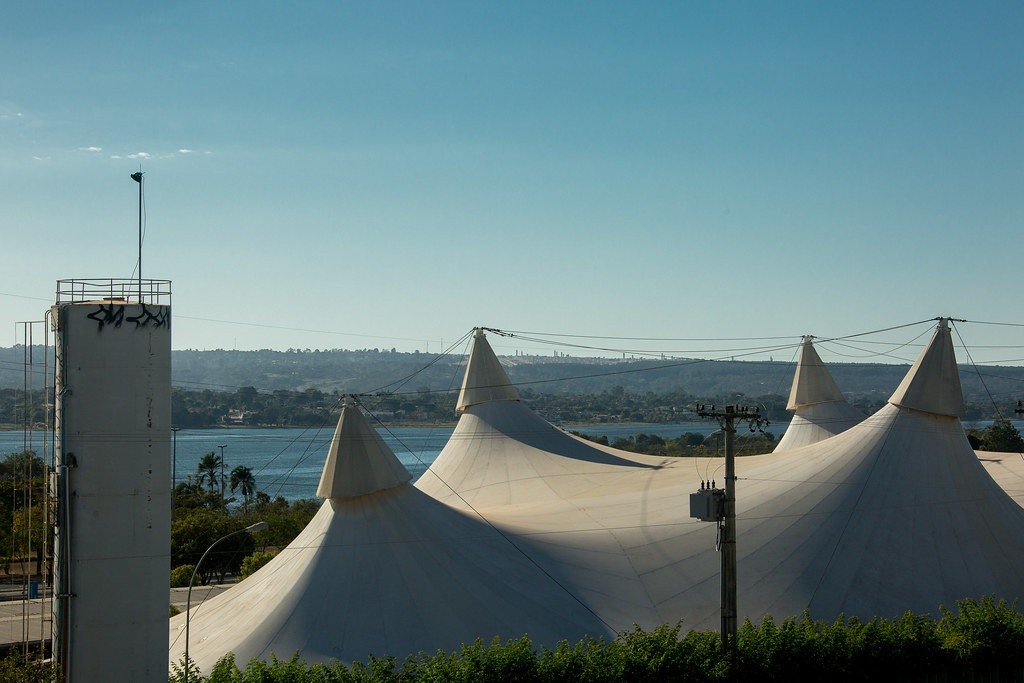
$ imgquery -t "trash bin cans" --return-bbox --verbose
[27,580,39,599]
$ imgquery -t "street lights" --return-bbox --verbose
[170,427,181,520]
[185,521,267,683]
[129,169,142,304]
[217,445,229,511]
